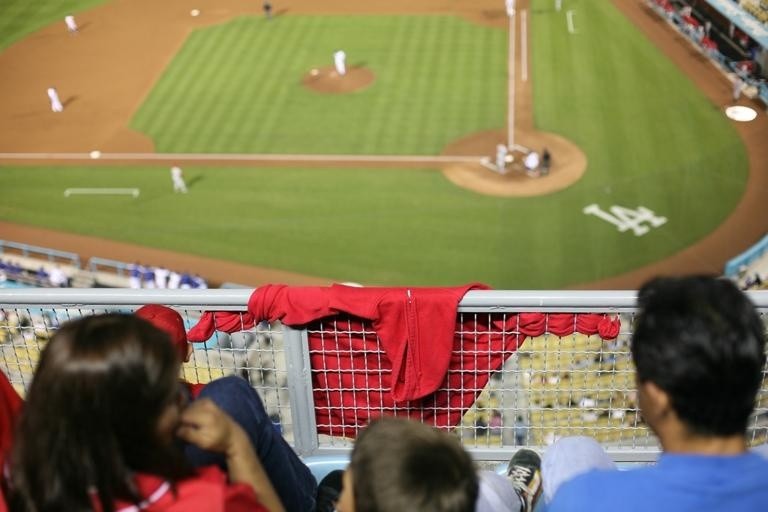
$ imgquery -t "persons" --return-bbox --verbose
[650,1,768,113]
[170,163,190,196]
[543,272,768,512]
[47,84,63,113]
[330,419,542,511]
[494,142,553,178]
[333,47,347,75]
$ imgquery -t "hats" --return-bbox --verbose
[133,304,187,358]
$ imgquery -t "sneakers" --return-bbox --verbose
[316,470,345,512]
[507,448,543,512]
